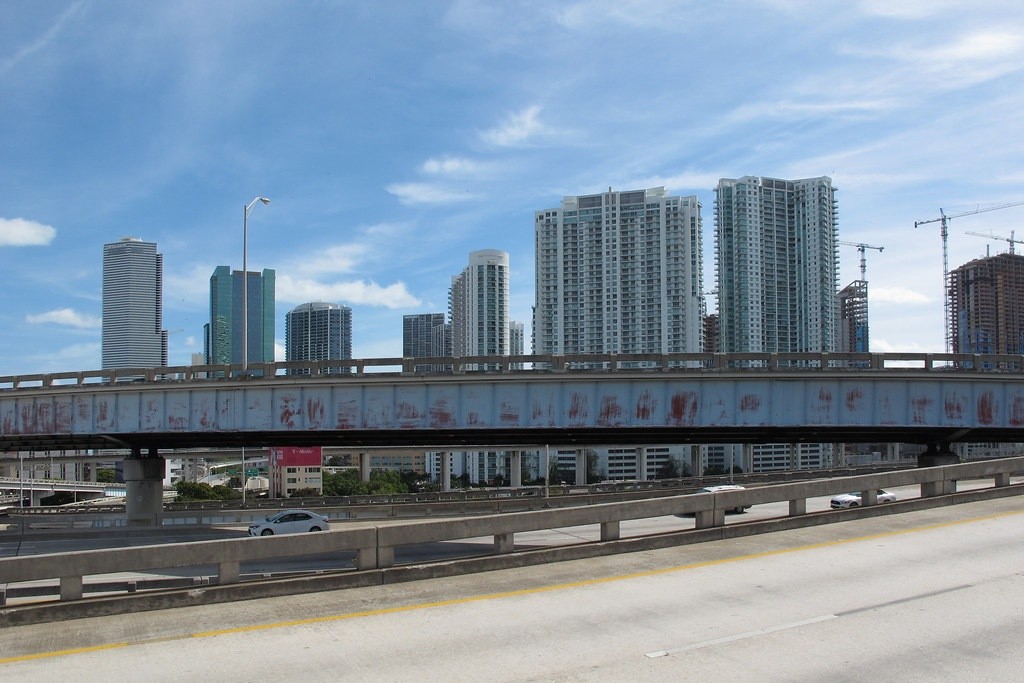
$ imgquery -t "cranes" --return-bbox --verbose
[839,239,884,281]
[914,200,1024,369]
[964,228,1024,254]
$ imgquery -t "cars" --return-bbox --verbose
[248,508,331,537]
[830,487,897,510]
[688,483,753,514]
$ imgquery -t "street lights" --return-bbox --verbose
[243,196,271,381]
